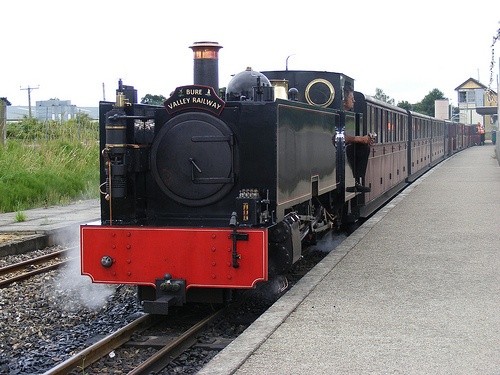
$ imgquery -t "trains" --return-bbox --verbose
[80,40,481,320]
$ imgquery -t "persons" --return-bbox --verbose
[343,87,358,110]
[476,122,485,144]
